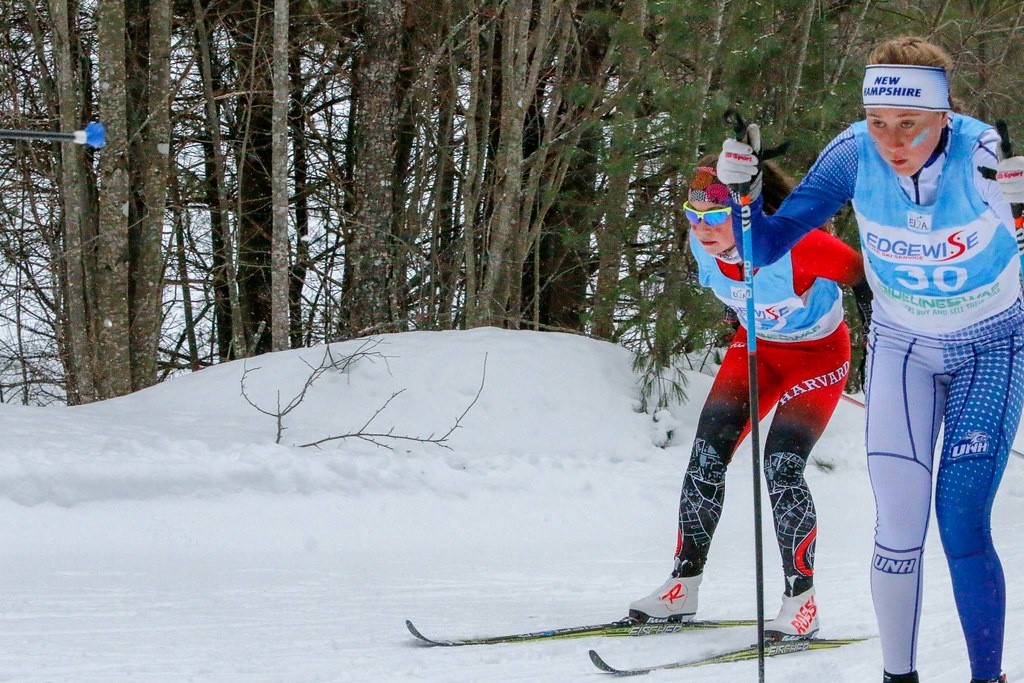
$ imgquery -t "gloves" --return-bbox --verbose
[996,134,1024,204]
[716,124,763,205]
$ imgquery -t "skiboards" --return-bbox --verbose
[404,616,871,675]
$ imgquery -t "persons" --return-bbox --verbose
[717,35,1024,683]
[630,152,873,641]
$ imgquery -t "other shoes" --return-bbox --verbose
[764,585,819,641]
[629,574,702,622]
[970,675,1008,683]
[883,670,919,683]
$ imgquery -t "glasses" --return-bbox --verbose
[683,201,733,226]
